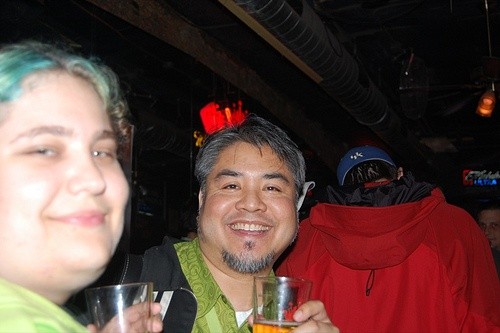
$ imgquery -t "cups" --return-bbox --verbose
[250,273,313,333]
[85,280,154,333]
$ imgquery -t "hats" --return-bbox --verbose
[338,146,397,187]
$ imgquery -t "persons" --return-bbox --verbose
[1,42,164,333]
[65,115,343,333]
[476,200,500,275]
[273,145,500,333]
